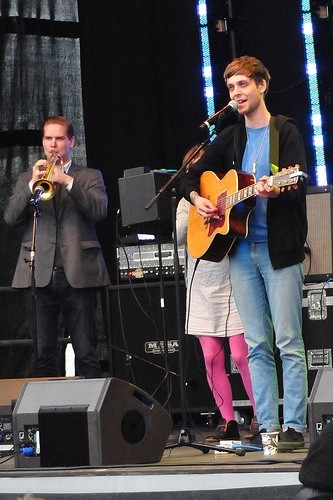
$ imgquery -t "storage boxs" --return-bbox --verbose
[224,280,333,433]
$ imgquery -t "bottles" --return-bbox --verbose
[64,336,75,377]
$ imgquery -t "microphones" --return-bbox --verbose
[184,377,193,387]
[199,100,239,131]
[29,186,44,207]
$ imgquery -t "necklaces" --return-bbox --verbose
[246,113,270,174]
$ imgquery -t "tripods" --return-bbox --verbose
[144,113,245,455]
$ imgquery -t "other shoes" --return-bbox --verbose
[286,427,305,447]
[206,420,241,441]
[250,428,281,445]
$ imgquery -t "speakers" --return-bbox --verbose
[118,171,180,227]
[307,368,333,446]
[299,185,333,283]
[12,377,174,469]
[106,280,219,414]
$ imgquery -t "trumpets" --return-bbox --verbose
[32,151,65,201]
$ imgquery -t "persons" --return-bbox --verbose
[172,145,259,442]
[178,56,307,452]
[4,116,111,378]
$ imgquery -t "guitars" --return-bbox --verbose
[187,164,308,263]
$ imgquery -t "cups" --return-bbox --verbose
[259,432,279,455]
